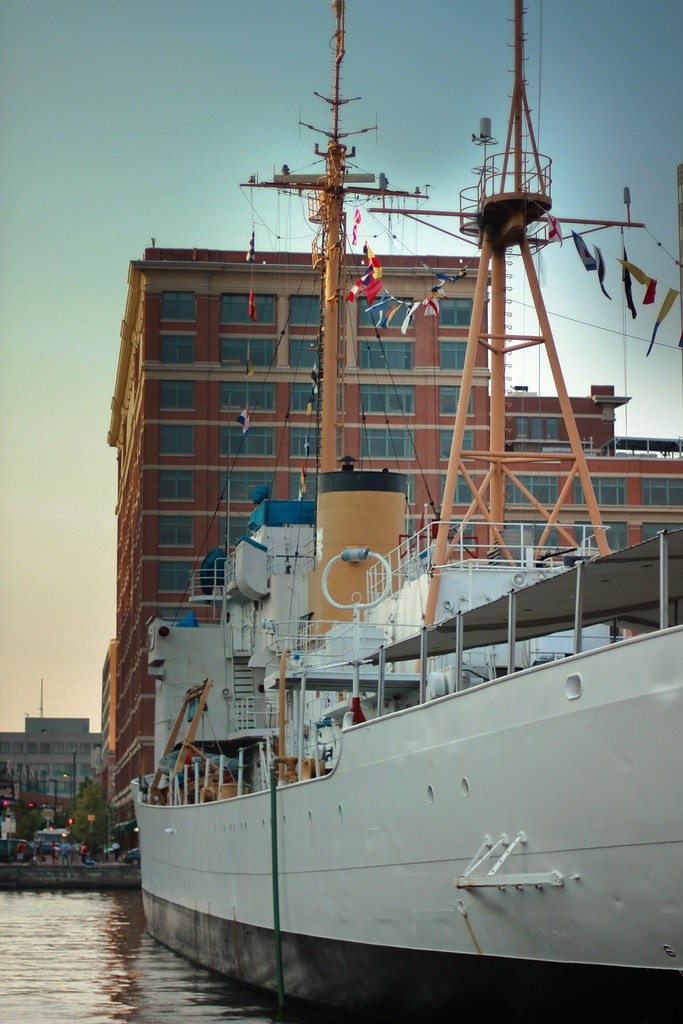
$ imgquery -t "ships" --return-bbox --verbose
[124,0,683,1024]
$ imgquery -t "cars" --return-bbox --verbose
[123,847,140,868]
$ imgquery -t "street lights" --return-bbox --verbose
[63,773,72,815]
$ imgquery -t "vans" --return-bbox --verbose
[0,839,34,864]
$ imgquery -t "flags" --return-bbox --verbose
[345,207,468,336]
[297,362,318,500]
[548,214,658,319]
[236,231,254,433]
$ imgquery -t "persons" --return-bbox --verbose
[15,837,88,865]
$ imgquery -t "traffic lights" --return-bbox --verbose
[68,817,74,825]
